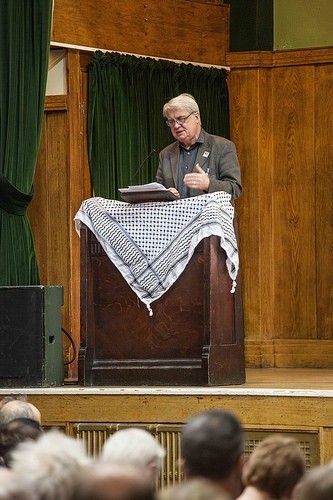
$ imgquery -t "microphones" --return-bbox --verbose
[125,148,157,188]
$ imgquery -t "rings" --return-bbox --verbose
[193,181,195,185]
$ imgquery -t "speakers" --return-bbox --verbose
[0,284,65,389]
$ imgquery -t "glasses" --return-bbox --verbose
[166,111,196,127]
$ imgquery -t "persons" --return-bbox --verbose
[154,93,242,203]
[0,399,333,500]
